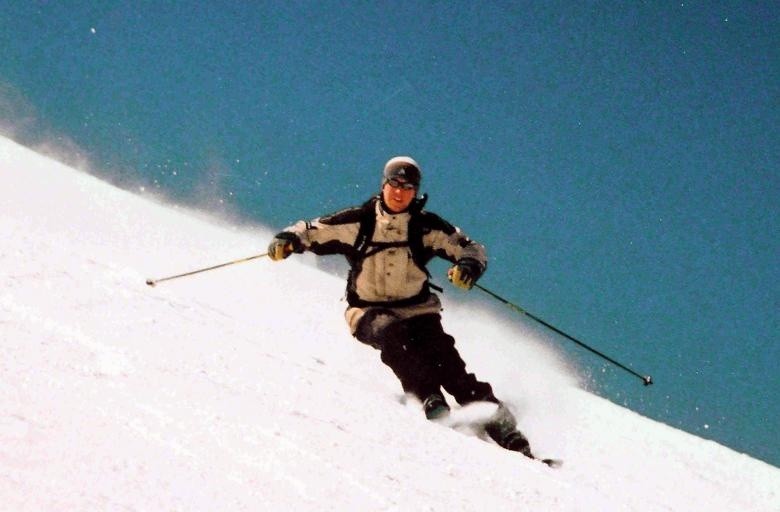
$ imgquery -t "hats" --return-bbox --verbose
[382,157,420,190]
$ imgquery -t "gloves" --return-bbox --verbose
[268,233,304,260]
[447,258,484,290]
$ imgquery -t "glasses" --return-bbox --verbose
[386,177,414,190]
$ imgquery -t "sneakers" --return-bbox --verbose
[420,391,449,419]
[486,418,529,453]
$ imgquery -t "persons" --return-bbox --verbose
[268,155,530,455]
